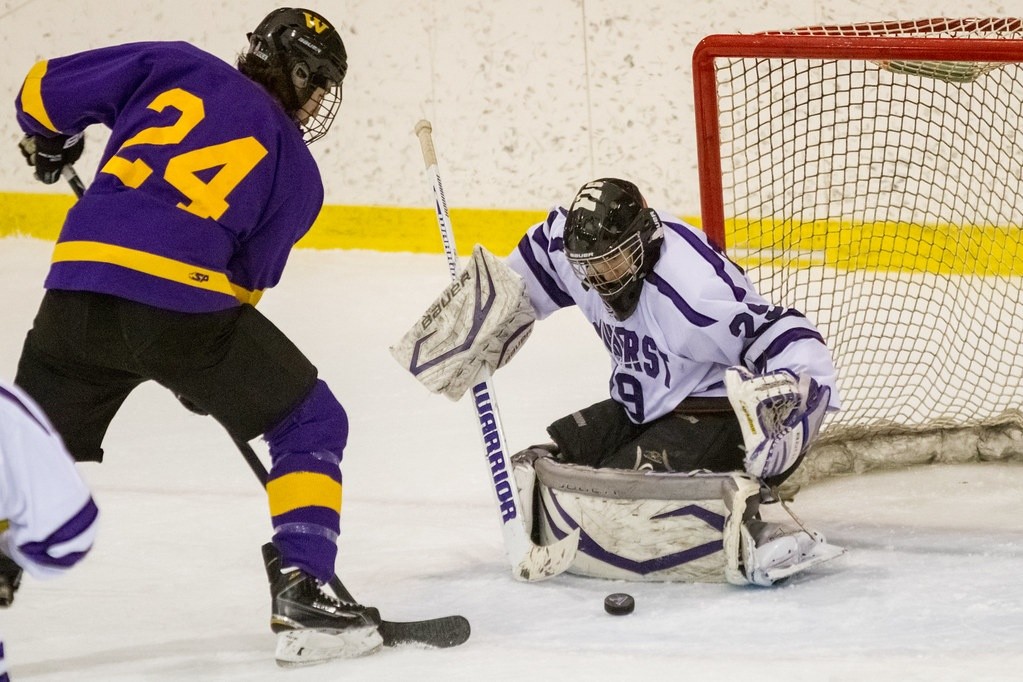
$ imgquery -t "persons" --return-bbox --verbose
[0,8,382,669]
[392,178,841,490]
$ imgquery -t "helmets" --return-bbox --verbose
[563,177,665,313]
[245,7,347,147]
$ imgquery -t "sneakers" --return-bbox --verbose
[0,549,24,610]
[261,542,385,667]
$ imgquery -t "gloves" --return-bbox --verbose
[18,130,85,185]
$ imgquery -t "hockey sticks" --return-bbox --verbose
[58,165,473,651]
[412,118,580,581]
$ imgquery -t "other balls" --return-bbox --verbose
[604,593,635,615]
